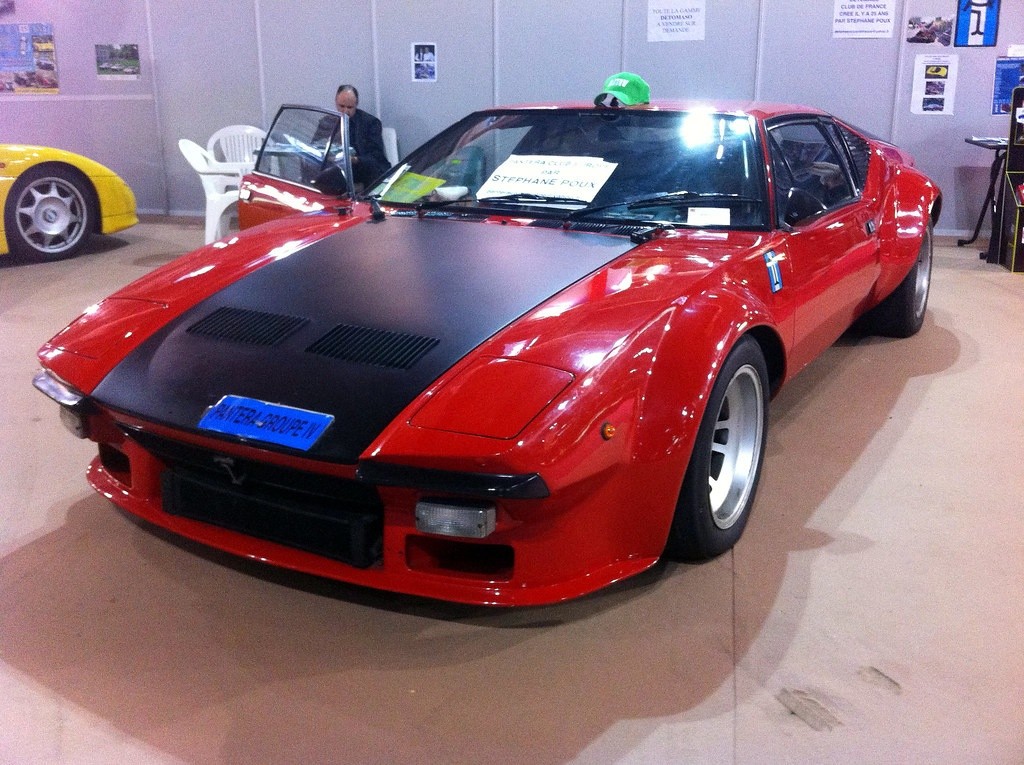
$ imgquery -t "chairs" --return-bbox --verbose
[178,124,280,246]
[353,127,399,193]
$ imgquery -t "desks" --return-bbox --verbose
[957,138,1009,259]
[253,143,353,186]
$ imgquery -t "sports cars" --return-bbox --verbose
[0,143,138,262]
[30,101,947,608]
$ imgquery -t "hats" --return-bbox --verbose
[594,72,650,106]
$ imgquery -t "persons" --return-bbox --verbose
[310,85,391,193]
[415,46,434,61]
